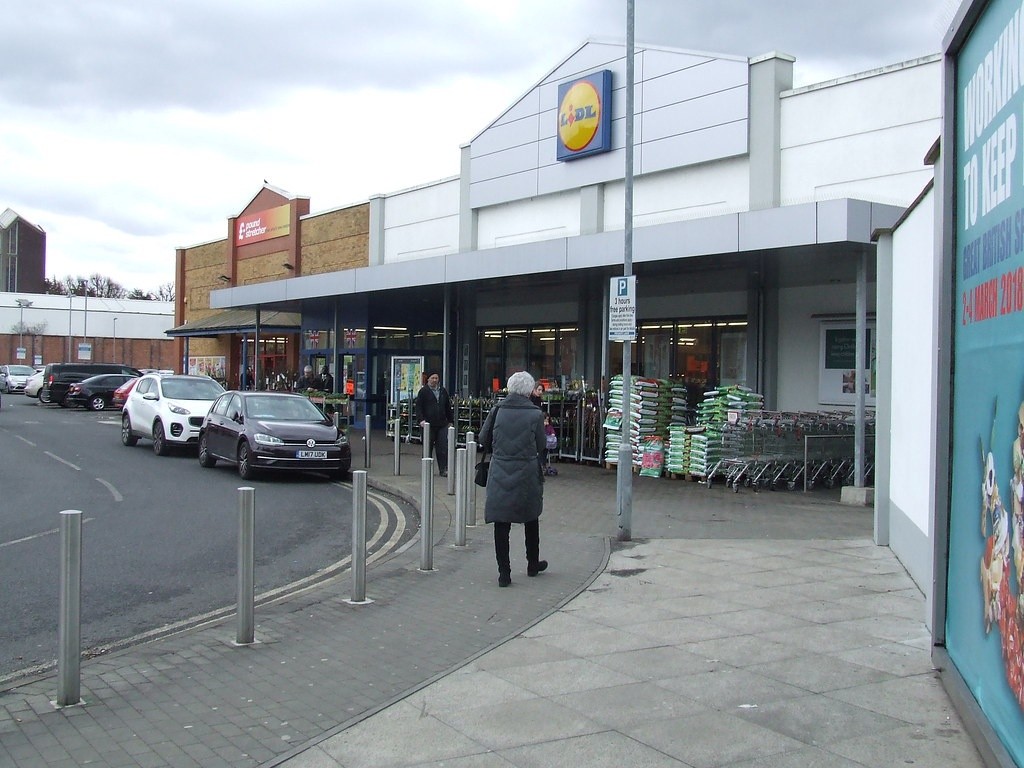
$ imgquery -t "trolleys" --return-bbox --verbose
[705,409,876,493]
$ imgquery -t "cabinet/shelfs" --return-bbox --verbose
[386,376,604,467]
[291,391,350,441]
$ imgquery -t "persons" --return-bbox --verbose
[296,365,324,394]
[478,370,548,587]
[538,413,556,471]
[319,366,333,414]
[415,369,454,477]
[529,381,545,409]
[240,366,255,391]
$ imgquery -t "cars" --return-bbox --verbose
[26,363,174,411]
[0,365,38,394]
[199,391,351,482]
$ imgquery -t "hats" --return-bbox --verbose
[423,368,441,379]
[320,366,329,375]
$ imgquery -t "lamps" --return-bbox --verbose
[218,275,231,282]
[282,263,294,270]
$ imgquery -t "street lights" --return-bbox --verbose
[66,295,75,363]
[114,317,119,362]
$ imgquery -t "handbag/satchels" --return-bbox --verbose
[473,462,491,488]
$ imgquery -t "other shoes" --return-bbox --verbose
[498,573,512,587]
[440,472,447,477]
[527,560,548,577]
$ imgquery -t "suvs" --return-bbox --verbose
[120,374,227,458]
